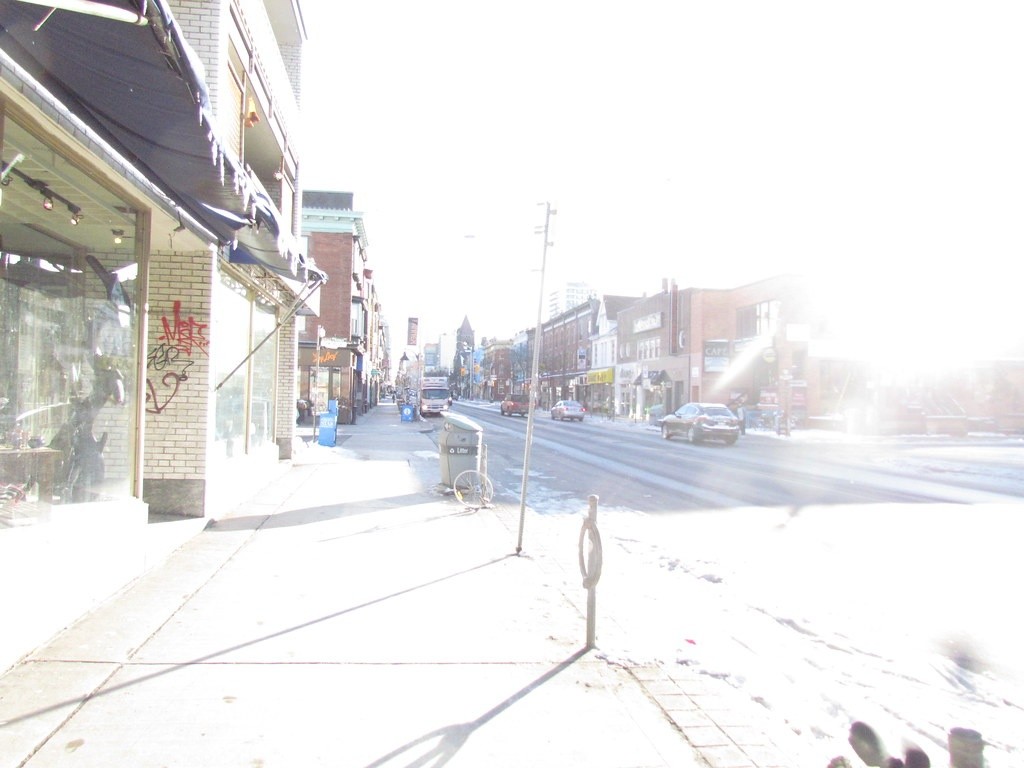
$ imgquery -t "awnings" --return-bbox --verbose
[1,0,328,388]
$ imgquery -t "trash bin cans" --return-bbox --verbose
[318,399,338,447]
[436,410,484,489]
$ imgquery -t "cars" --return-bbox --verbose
[550,400,585,421]
[500,393,530,416]
[662,403,739,447]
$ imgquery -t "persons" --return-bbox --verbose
[392,394,396,403]
[737,402,747,435]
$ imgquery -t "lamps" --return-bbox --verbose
[43,196,54,210]
[111,229,124,244]
[71,212,84,225]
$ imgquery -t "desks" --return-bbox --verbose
[0,445,61,521]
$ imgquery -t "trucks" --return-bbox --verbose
[419,377,451,418]
